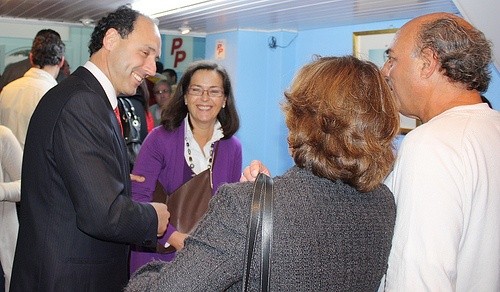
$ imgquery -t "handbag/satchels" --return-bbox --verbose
[153,169,211,254]
[119,97,142,165]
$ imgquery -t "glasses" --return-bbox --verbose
[153,89,169,94]
[187,85,223,98]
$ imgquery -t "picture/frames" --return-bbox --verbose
[352,28,423,136]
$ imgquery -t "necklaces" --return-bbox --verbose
[186,137,215,177]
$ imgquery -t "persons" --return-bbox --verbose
[122,55,397,292]
[0,124,24,292]
[378,12,500,292]
[128,59,243,278]
[7,5,171,292]
[0,28,178,157]
